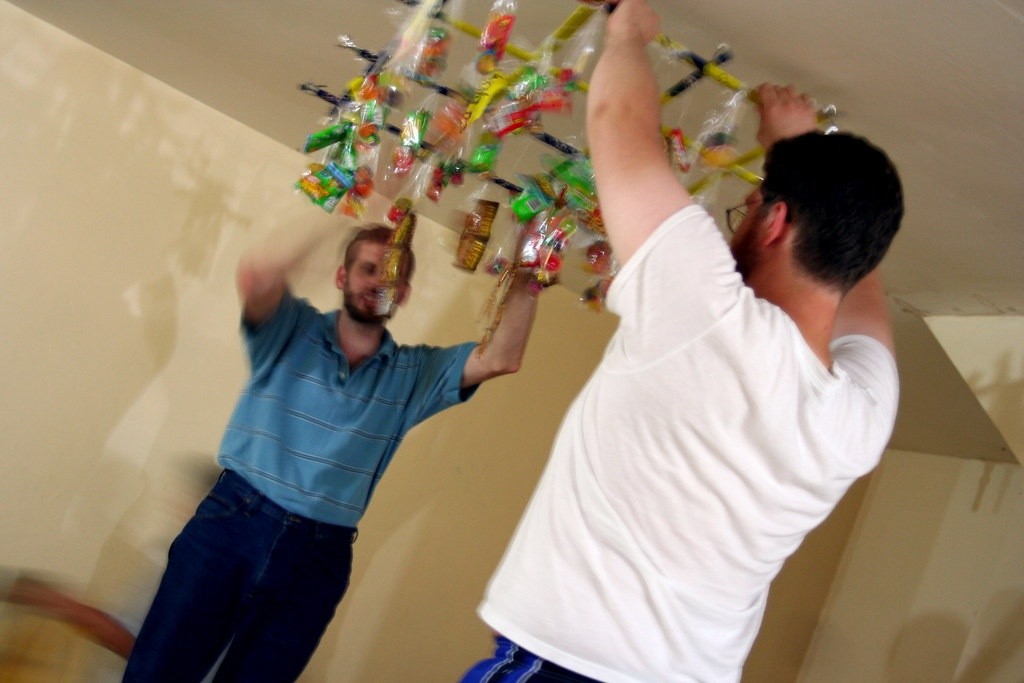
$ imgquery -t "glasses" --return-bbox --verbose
[725,201,754,233]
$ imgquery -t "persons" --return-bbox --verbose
[122,213,540,683]
[459,0,905,683]
